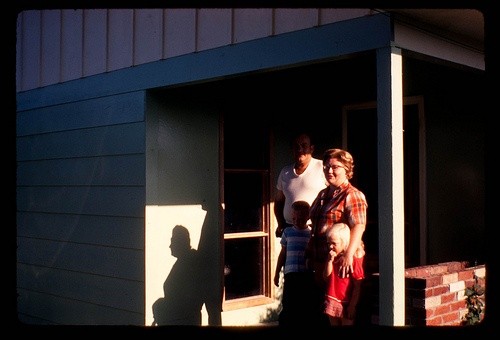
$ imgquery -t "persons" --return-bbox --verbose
[274,201,312,323]
[275,133,330,238]
[321,224,365,325]
[305,149,368,322]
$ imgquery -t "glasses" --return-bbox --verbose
[323,165,345,170]
